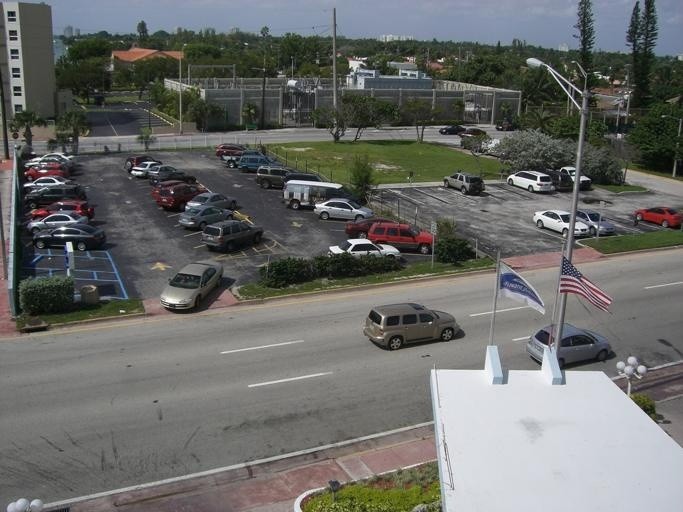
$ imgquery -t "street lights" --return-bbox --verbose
[242,42,265,129]
[614,355,647,396]
[179,44,188,135]
[525,49,591,383]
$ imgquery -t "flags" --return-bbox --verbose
[558,256,613,312]
[497,262,545,316]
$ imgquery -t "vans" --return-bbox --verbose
[281,180,343,210]
[506,171,552,195]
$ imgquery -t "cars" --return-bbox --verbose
[313,198,374,220]
[344,218,395,238]
[531,208,590,237]
[560,166,590,187]
[363,301,457,350]
[327,238,401,262]
[545,171,572,192]
[216,141,324,189]
[526,320,612,370]
[442,172,484,196]
[575,208,615,236]
[20,147,105,251]
[437,123,484,145]
[495,123,514,130]
[160,260,223,310]
[122,149,264,251]
[633,205,683,229]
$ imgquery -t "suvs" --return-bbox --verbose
[367,223,432,254]
